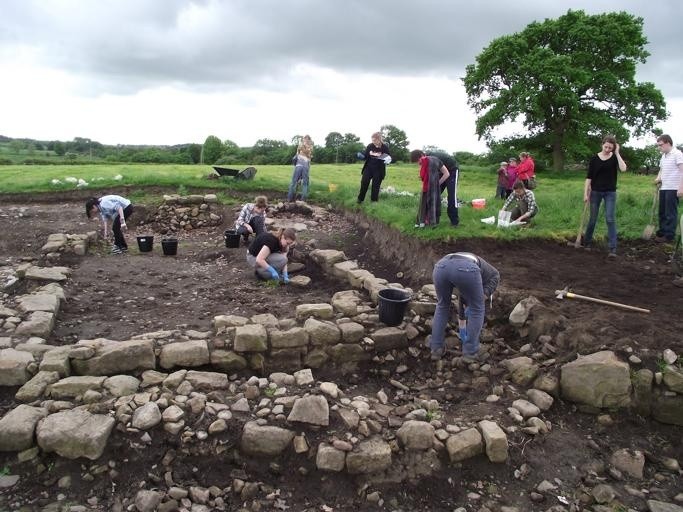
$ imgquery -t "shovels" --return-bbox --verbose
[642,182,662,241]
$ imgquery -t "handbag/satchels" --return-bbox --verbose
[525,178,535,189]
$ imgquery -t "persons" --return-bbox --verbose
[583,134,627,258]
[409,148,460,230]
[286,135,315,203]
[428,252,500,359]
[654,134,683,242]
[84,194,133,254]
[244,226,296,284]
[496,150,540,225]
[355,132,391,204]
[233,195,268,243]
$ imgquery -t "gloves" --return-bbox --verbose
[282,271,289,285]
[267,265,279,280]
[120,217,128,230]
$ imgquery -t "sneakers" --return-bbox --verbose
[431,348,447,361]
[608,252,617,258]
[414,223,426,228]
[654,235,673,244]
[462,355,479,364]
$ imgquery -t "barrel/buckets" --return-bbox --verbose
[136,235,154,252]
[377,288,411,326]
[328,183,339,193]
[161,239,177,255]
[225,231,241,248]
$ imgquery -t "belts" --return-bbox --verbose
[446,254,473,260]
